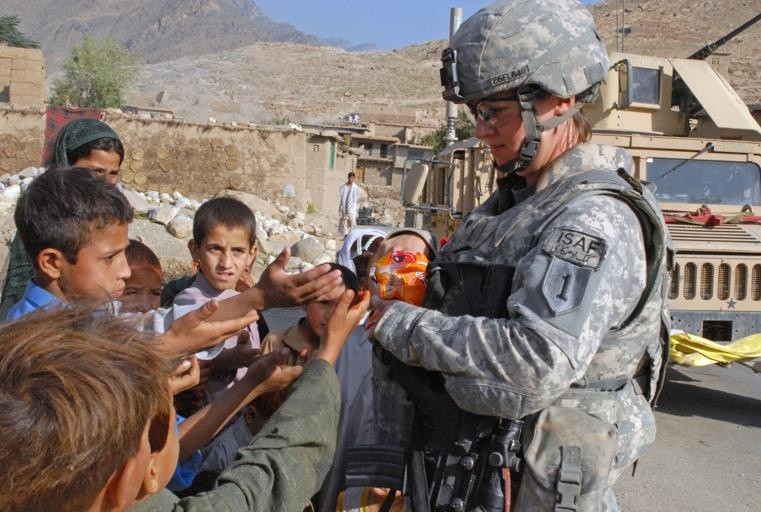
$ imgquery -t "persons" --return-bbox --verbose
[362,0,677,512]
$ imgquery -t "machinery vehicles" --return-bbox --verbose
[397,10,760,368]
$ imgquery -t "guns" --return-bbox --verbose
[371,265,524,512]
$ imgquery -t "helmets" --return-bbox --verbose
[439,0,610,105]
